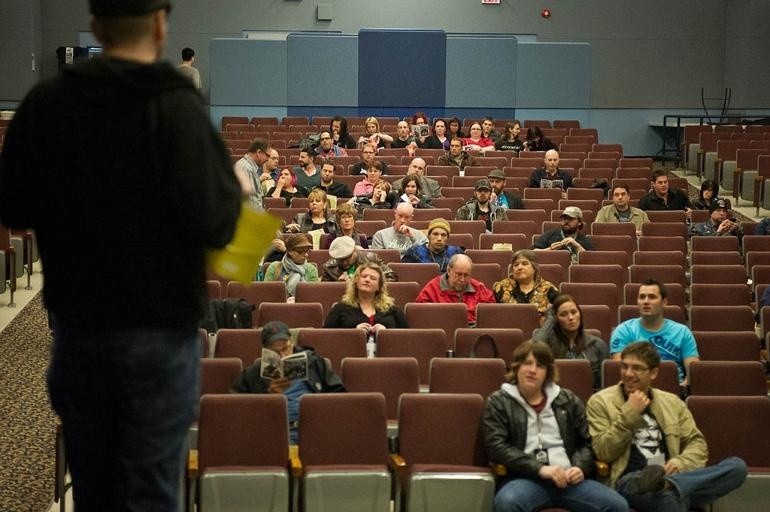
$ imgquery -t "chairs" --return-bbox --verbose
[1,229,42,309]
[2,110,19,146]
[184,110,769,511]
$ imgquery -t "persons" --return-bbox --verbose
[177,47,204,94]
[1,0,241,509]
[201,113,768,444]
[477,339,629,511]
[585,341,748,511]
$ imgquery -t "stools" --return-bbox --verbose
[701,87,733,124]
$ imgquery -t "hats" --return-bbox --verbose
[285,233,313,252]
[709,198,728,215]
[474,179,492,193]
[560,206,584,219]
[328,236,356,261]
[487,170,505,180]
[428,218,449,238]
[260,321,292,347]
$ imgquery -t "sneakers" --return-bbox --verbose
[628,465,665,495]
